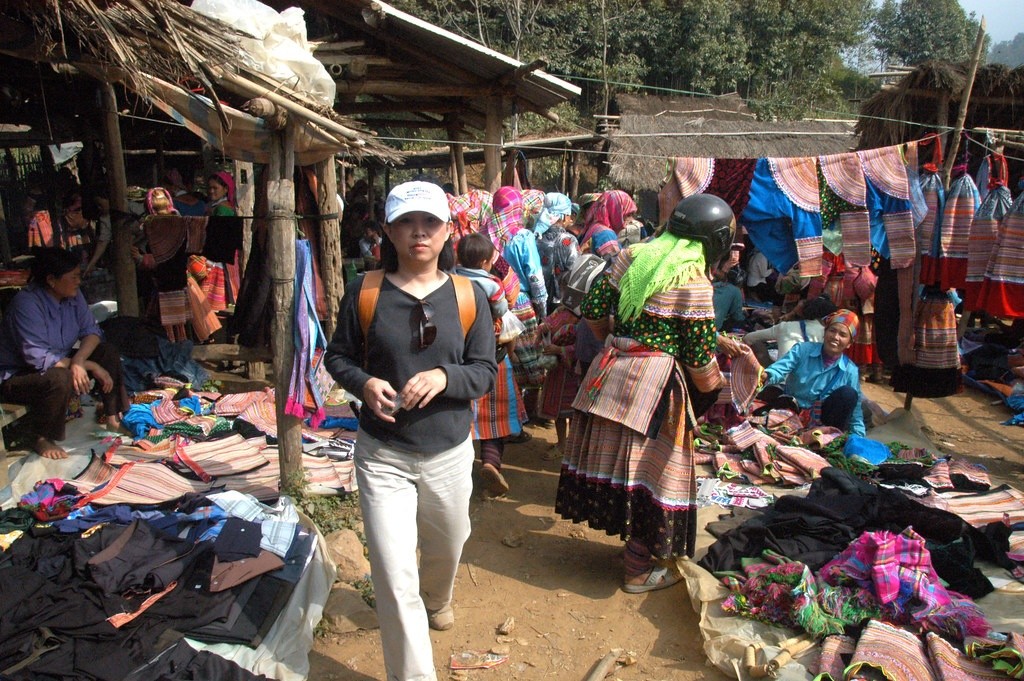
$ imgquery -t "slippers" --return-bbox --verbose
[624,566,682,593]
[543,447,565,460]
[481,467,508,495]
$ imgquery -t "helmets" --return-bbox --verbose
[667,192,735,264]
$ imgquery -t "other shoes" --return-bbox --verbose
[429,605,454,630]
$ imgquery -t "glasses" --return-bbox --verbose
[419,299,437,350]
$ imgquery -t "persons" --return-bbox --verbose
[83,182,148,279]
[323,181,498,681]
[372,187,386,227]
[759,309,867,443]
[747,248,774,299]
[577,190,650,261]
[19,168,97,247]
[581,194,750,594]
[0,247,136,460]
[743,300,830,360]
[447,187,582,496]
[203,170,241,345]
[341,180,370,258]
[775,248,886,384]
[130,185,188,343]
[242,224,271,350]
[714,334,750,360]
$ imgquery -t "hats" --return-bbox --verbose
[384,181,451,224]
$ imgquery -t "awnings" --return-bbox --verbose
[262,1,582,196]
